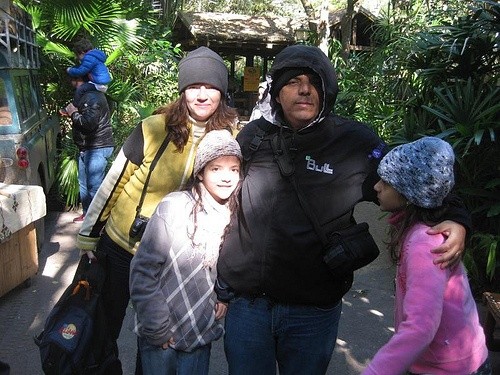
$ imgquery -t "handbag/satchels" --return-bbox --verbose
[95,358,122,375]
[319,222,380,279]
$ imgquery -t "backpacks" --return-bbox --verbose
[39,251,105,375]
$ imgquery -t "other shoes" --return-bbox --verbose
[73,215,86,224]
[59,109,69,117]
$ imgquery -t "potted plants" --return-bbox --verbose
[421,79,500,291]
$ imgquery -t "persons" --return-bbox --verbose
[61,64,114,223]
[215,46,470,375]
[353,137,493,375]
[250,82,274,122]
[128,129,243,375]
[76,47,243,375]
[60,40,112,119]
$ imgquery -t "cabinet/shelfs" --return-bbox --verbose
[0,218,38,299]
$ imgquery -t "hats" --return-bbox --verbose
[193,129,243,179]
[178,46,228,97]
[272,67,313,103]
[377,136,456,208]
